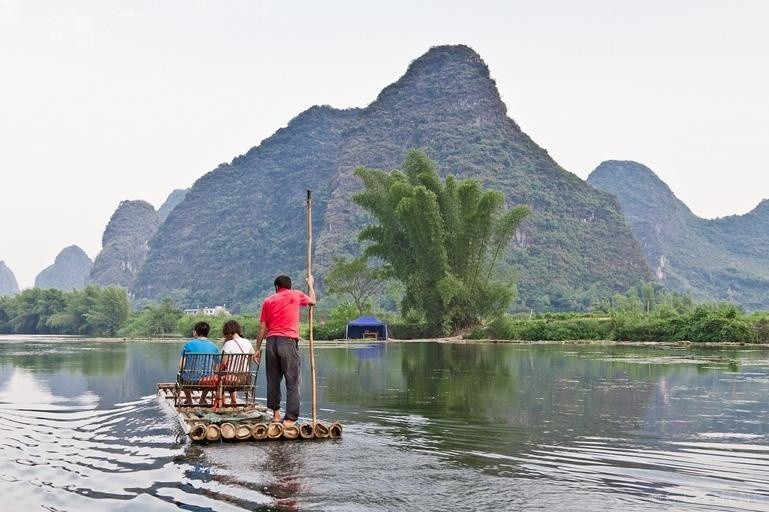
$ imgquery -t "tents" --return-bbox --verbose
[346,315,388,341]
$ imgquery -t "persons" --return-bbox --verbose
[253,274,316,424]
[220,320,255,405]
[179,321,221,404]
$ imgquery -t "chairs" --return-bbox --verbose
[215,349,262,405]
[171,347,219,408]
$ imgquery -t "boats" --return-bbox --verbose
[157,380,344,443]
